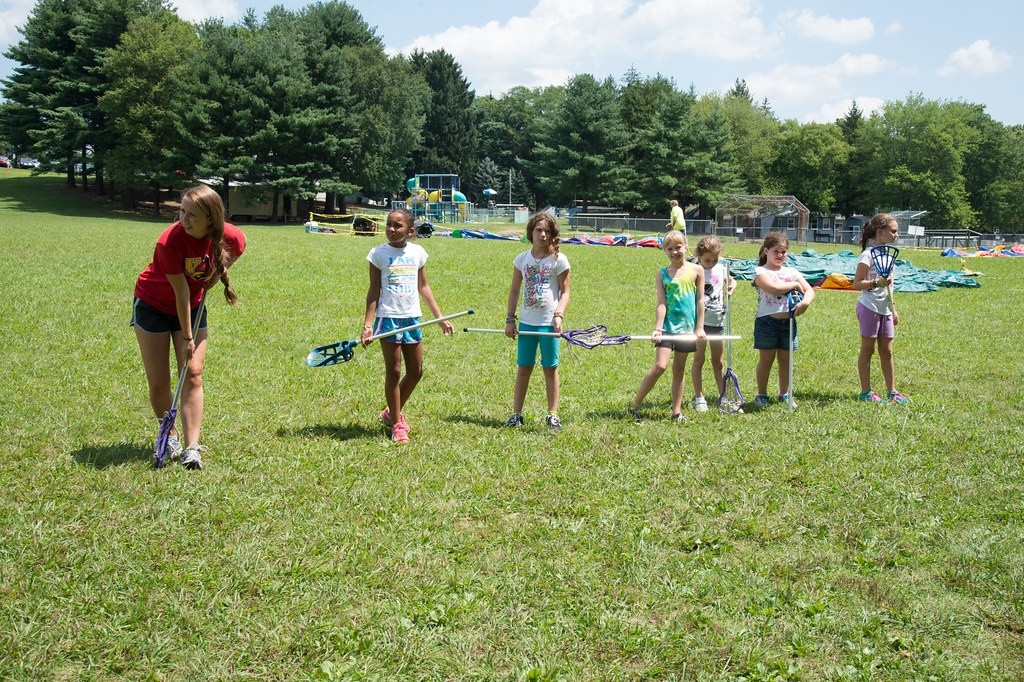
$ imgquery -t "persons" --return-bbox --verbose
[692,235,737,413]
[360,208,455,443]
[745,226,816,412]
[501,212,573,433]
[7,156,12,169]
[16,156,21,169]
[666,201,687,236]
[630,230,706,426]
[487,198,497,211]
[851,212,912,404]
[128,185,245,469]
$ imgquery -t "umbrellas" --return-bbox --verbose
[484,187,497,199]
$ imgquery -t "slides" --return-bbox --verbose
[406,177,429,221]
[453,191,470,222]
[429,189,442,221]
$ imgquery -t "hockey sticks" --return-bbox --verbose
[153,287,208,468]
[600,334,742,345]
[463,325,608,350]
[870,245,900,316]
[786,290,804,412]
[305,309,475,368]
[716,260,745,415]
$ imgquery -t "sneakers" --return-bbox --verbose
[777,394,797,409]
[546,413,562,430]
[380,407,410,434]
[671,413,694,423]
[755,395,768,408]
[693,401,709,414]
[859,390,888,404]
[165,435,183,458]
[720,399,744,414]
[182,443,210,470]
[391,423,410,443]
[887,390,909,403]
[628,408,644,425]
[503,414,524,427]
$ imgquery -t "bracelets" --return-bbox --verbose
[183,338,193,341]
[363,325,372,329]
[880,277,885,288]
[655,328,663,332]
[553,311,563,319]
[504,312,518,324]
[873,278,877,288]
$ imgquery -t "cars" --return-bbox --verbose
[0,155,12,168]
[16,157,96,175]
